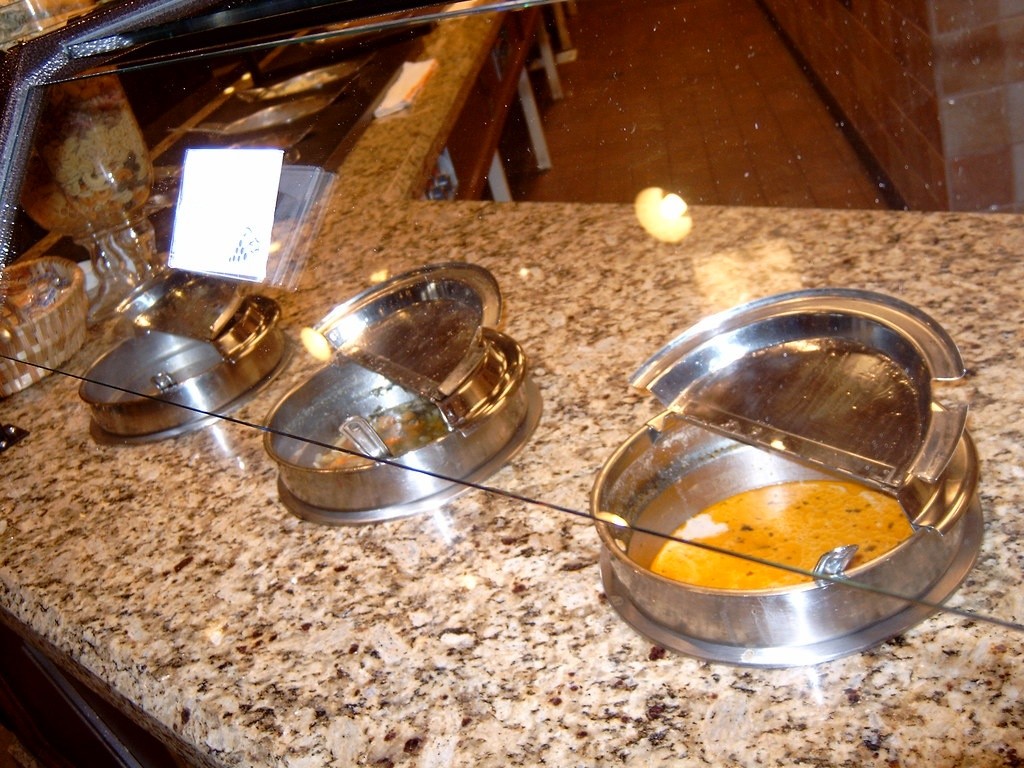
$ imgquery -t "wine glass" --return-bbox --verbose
[16,61,168,333]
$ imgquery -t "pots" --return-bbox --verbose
[77,269,286,436]
[590,287,980,648]
[261,261,530,512]
